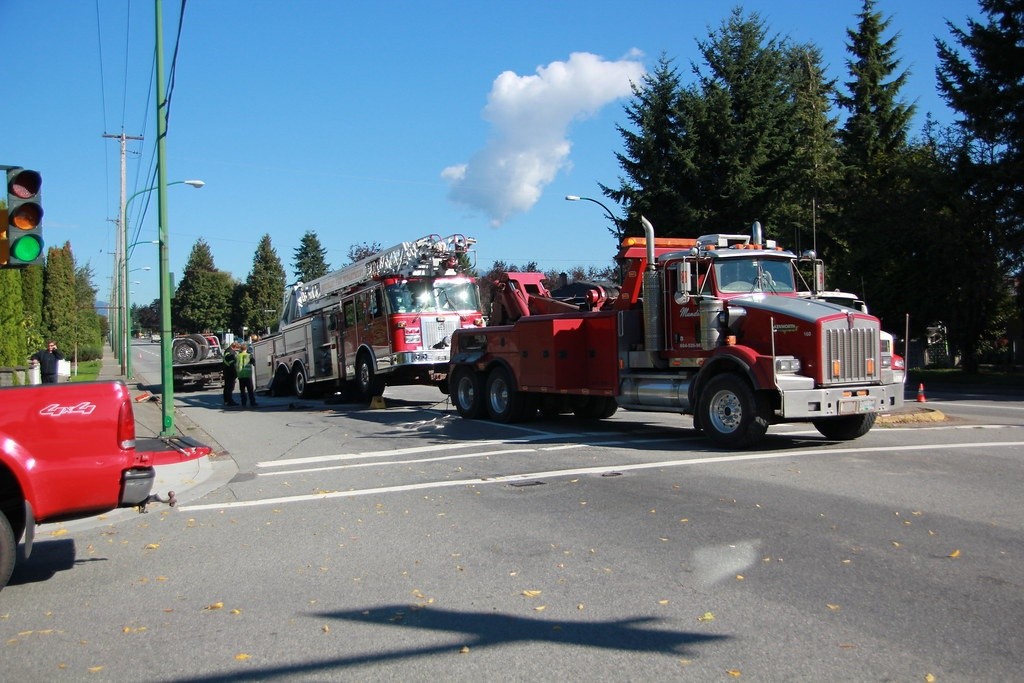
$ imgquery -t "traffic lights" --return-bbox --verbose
[4,167,46,270]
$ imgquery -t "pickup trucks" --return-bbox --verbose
[0,381,177,594]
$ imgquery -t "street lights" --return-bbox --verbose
[109,180,204,378]
[566,194,625,288]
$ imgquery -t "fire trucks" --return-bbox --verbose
[251,232,485,399]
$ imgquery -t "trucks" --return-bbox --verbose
[172,333,225,390]
[445,215,905,448]
[150,335,161,343]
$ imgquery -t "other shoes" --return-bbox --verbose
[224,400,234,406]
[252,403,258,406]
[232,400,239,405]
[242,403,246,406]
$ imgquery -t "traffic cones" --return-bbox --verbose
[918,382,927,402]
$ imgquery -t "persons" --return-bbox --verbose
[222,342,241,406]
[30,340,64,384]
[234,343,258,406]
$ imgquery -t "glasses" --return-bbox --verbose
[48,345,54,347]
